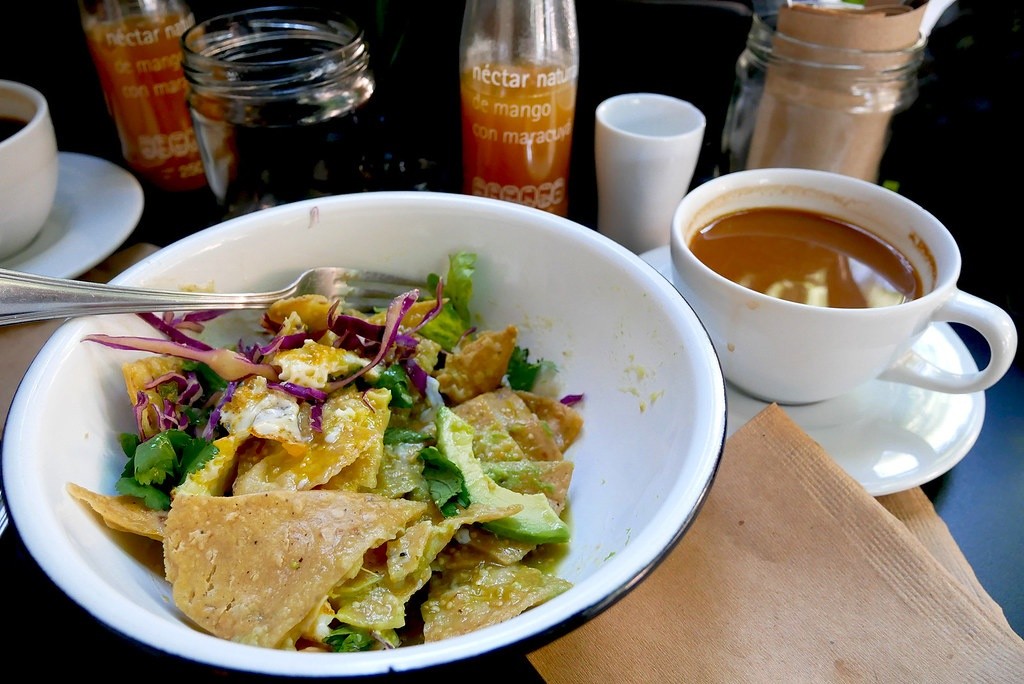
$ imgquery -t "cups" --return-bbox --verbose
[664,167,1019,408]
[592,93,705,258]
[1,79,60,264]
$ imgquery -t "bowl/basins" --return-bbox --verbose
[1,188,728,680]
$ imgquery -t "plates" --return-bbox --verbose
[3,151,146,286]
[638,245,987,495]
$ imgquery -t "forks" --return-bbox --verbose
[1,270,433,326]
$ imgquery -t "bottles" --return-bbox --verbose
[74,1,233,197]
[457,0,581,217]
[176,8,392,221]
[719,9,930,181]
